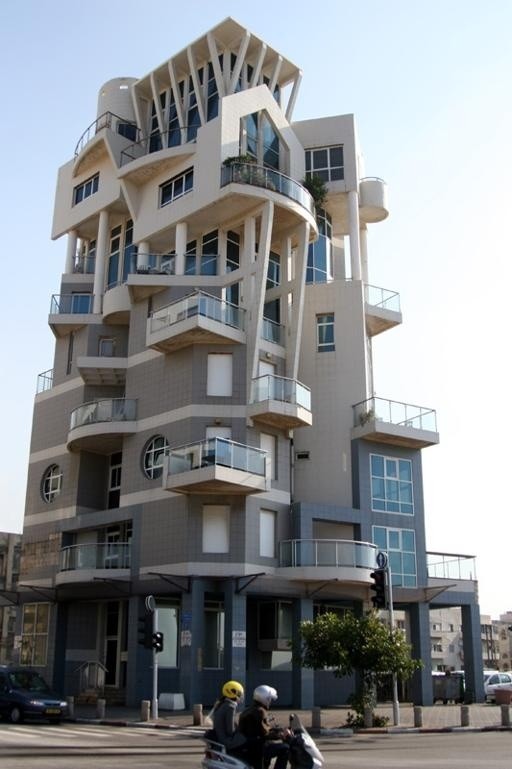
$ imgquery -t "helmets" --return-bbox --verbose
[253,685,278,710]
[223,681,244,704]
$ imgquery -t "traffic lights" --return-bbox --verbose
[137,611,154,650]
[153,632,163,651]
[370,570,387,610]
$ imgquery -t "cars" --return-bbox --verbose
[432,668,512,704]
[0,665,69,725]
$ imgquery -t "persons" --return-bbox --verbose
[213,680,271,769]
[239,685,293,769]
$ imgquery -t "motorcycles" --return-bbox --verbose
[200,711,325,769]
